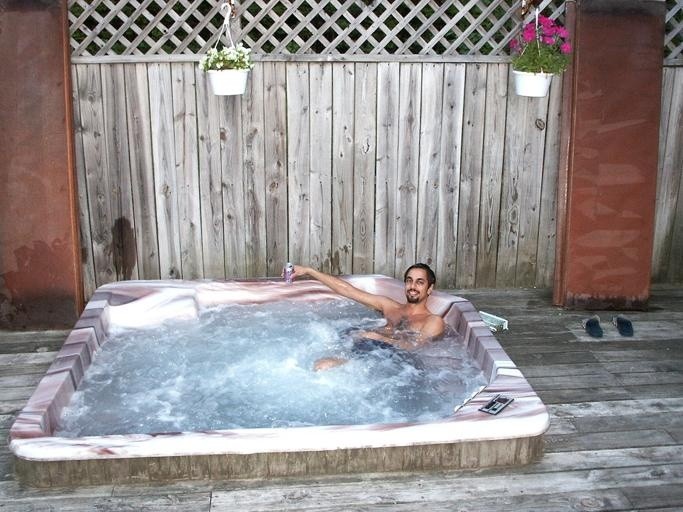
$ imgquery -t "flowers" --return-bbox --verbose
[506,16,573,77]
[198,42,254,73]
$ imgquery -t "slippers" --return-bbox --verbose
[611,313,633,337]
[582,313,604,338]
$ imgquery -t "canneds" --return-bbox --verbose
[283,263,293,285]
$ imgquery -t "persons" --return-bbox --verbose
[280,257,447,383]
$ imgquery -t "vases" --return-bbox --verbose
[207,70,248,96]
[511,70,554,98]
